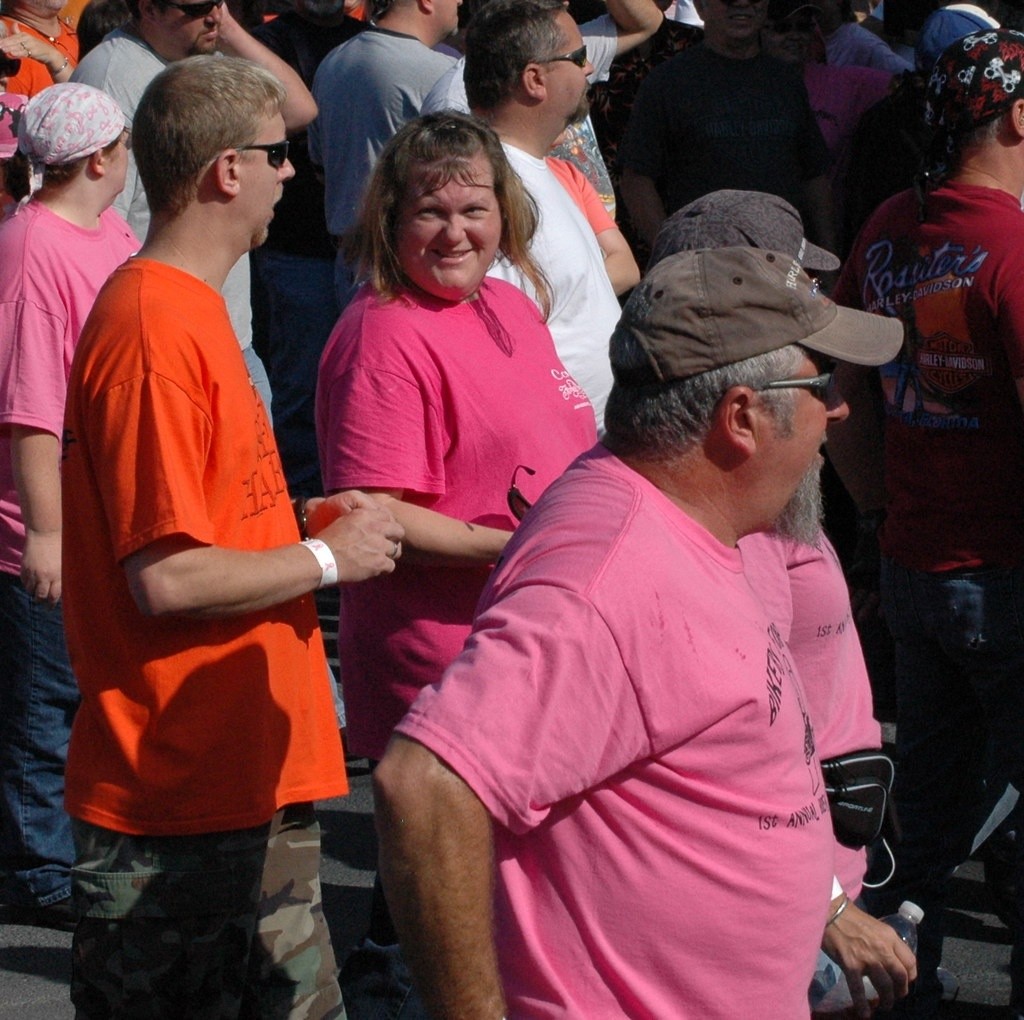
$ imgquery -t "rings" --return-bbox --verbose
[390,540,398,558]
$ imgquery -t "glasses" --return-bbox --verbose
[239,140,289,168]
[162,0,223,17]
[518,45,588,85]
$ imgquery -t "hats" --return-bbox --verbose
[919,3,1000,73]
[918,29,1024,144]
[645,190,840,279]
[609,246,904,395]
[18,83,125,163]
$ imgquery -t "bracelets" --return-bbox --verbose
[299,538,340,589]
[823,898,851,928]
[295,495,312,538]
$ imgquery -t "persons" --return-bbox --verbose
[377,245,905,1020]
[61,47,404,1019]
[312,100,606,997]
[0,0,1022,1020]
[0,82,153,922]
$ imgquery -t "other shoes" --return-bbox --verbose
[17,897,76,930]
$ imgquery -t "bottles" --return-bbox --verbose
[810,899,924,1020]
